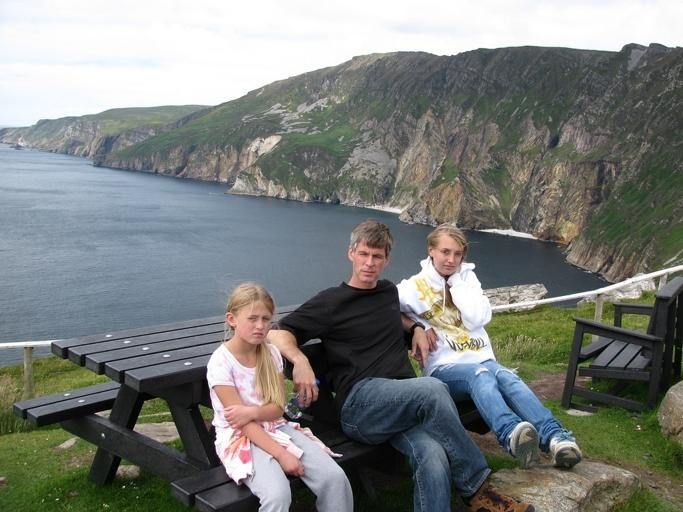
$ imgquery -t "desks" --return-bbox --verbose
[51,302,419,392]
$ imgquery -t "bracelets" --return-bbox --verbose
[410,321,425,336]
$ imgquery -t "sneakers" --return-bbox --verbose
[549,430,583,470]
[466,480,535,512]
[510,421,540,469]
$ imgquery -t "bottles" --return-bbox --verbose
[284,378,320,420]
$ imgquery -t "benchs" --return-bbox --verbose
[169,380,481,510]
[11,380,149,426]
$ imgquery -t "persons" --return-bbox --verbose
[392,222,584,473]
[204,282,354,510]
[263,218,538,511]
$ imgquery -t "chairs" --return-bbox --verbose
[561,278,683,415]
